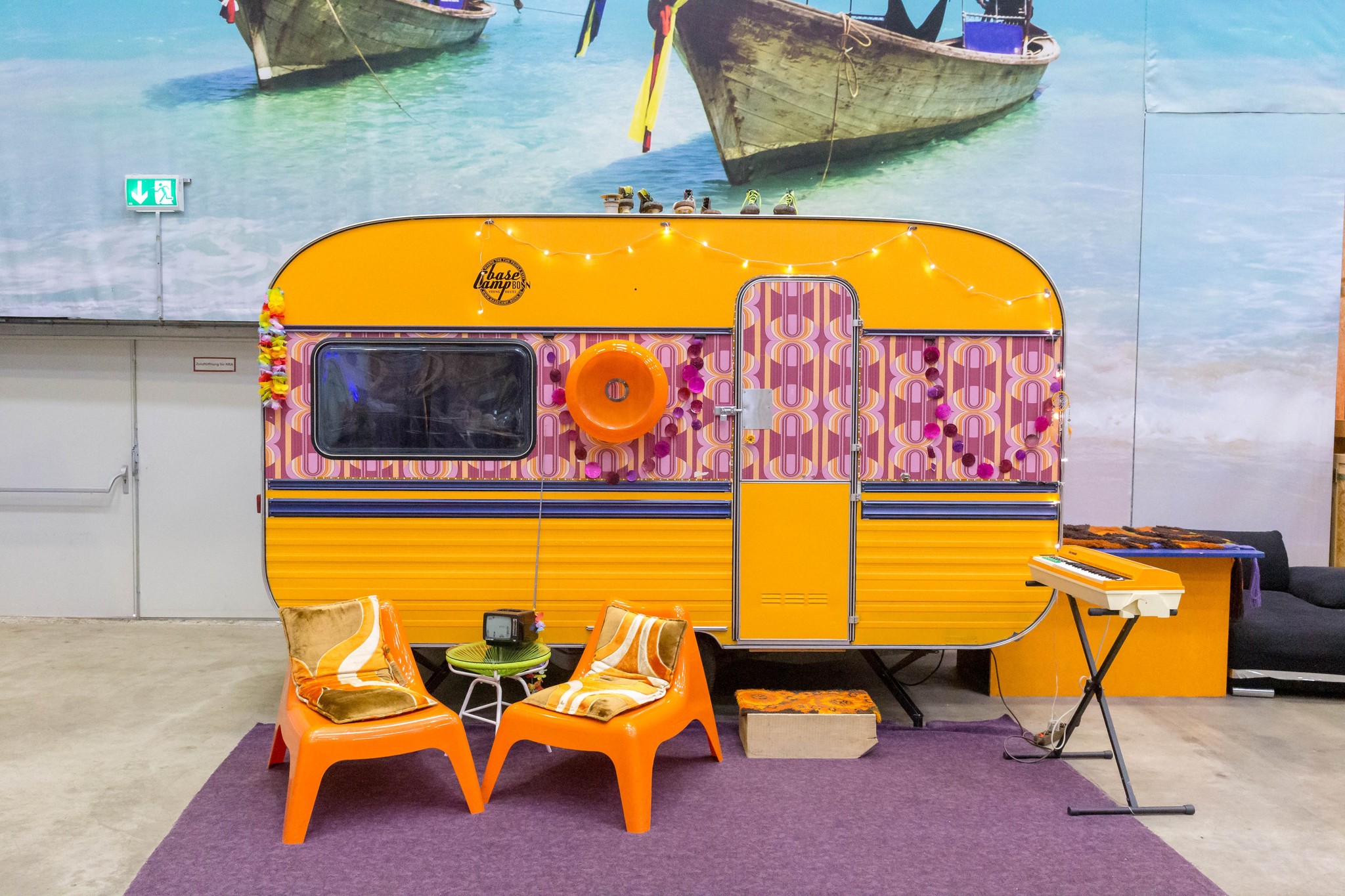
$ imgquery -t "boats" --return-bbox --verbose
[219,0,497,90]
[646,0,1061,187]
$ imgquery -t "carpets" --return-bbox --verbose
[122,720,1229,896]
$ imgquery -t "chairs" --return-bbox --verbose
[265,594,486,847]
[483,595,725,836]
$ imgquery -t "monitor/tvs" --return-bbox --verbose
[483,609,538,647]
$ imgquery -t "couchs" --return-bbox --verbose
[1184,527,1345,700]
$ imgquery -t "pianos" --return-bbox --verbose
[1001,544,1197,817]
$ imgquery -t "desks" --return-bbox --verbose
[444,636,555,754]
[954,524,1268,699]
